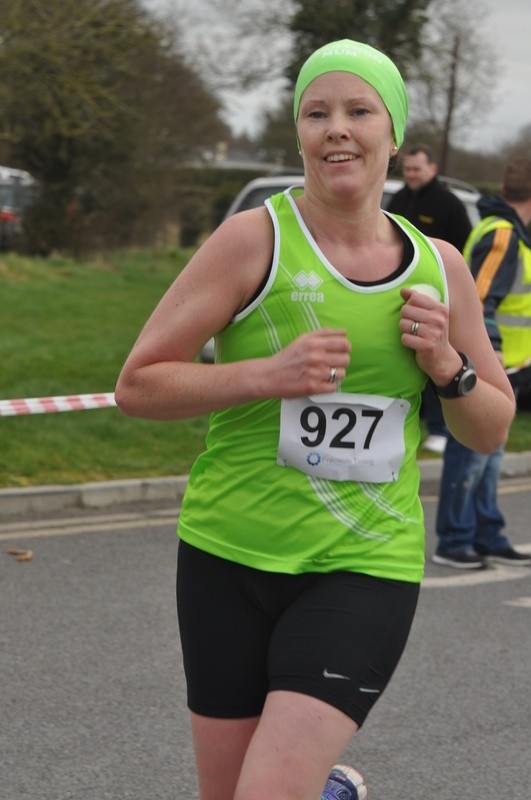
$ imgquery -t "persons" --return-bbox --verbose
[382,146,530,572]
[114,38,520,800]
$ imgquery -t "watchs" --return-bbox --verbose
[435,350,479,399]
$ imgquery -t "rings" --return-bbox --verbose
[327,368,336,382]
[412,321,421,336]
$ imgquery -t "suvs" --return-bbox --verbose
[199,170,481,363]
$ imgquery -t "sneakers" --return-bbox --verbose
[431,544,484,569]
[478,544,531,565]
[319,764,367,800]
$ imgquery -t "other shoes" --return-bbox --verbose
[422,433,446,453]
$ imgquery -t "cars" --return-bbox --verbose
[0,167,41,249]
